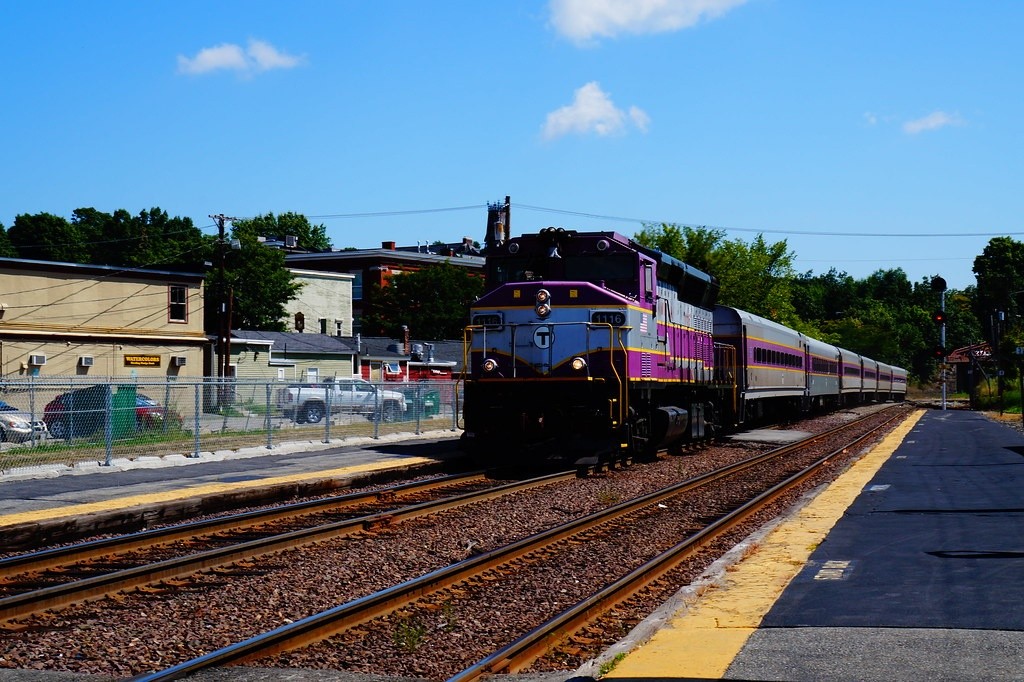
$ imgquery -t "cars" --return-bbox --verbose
[0,401,45,444]
[45,387,182,445]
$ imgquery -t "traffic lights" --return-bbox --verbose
[933,345,947,360]
[933,311,947,326]
[932,277,946,293]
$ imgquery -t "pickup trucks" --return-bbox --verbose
[276,376,407,427]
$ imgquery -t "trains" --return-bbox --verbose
[456,228,911,482]
